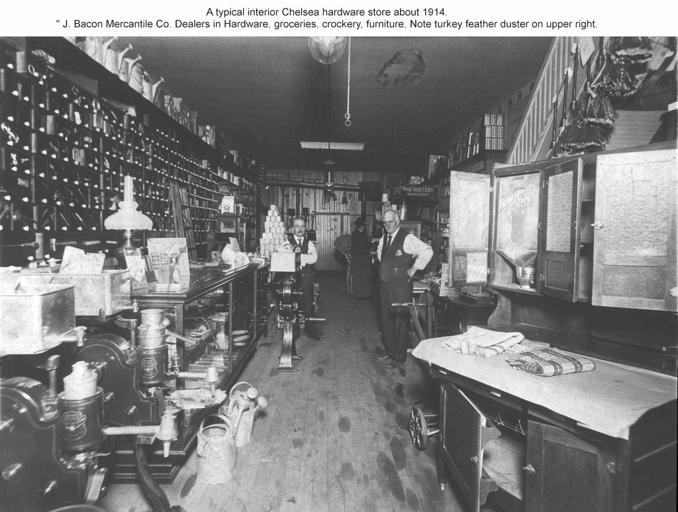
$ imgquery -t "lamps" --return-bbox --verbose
[103,175,155,253]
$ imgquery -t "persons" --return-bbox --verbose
[377,210,434,369]
[287,217,320,341]
[352,224,370,262]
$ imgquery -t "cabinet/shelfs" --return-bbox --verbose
[108,261,271,482]
[0,37,215,266]
[411,140,678,512]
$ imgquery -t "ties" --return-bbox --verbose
[298,239,301,246]
[388,236,392,247]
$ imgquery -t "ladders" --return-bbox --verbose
[169,181,198,262]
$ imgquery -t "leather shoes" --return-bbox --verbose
[377,353,401,368]
